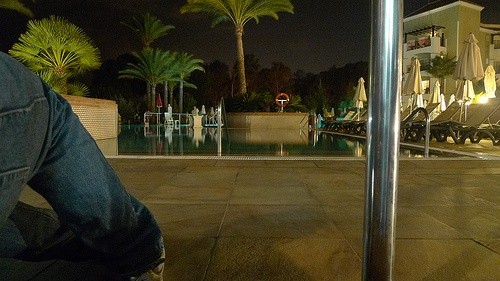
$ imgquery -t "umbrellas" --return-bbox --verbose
[353,77,367,122]
[155,94,163,113]
[403,57,423,115]
[452,32,485,102]
[408,64,496,113]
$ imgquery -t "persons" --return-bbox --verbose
[0,48,166,281]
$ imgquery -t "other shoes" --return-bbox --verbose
[130,235,165,281]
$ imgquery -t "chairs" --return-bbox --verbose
[320,98,500,147]
[164,113,174,125]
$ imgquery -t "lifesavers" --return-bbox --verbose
[276,92,290,107]
